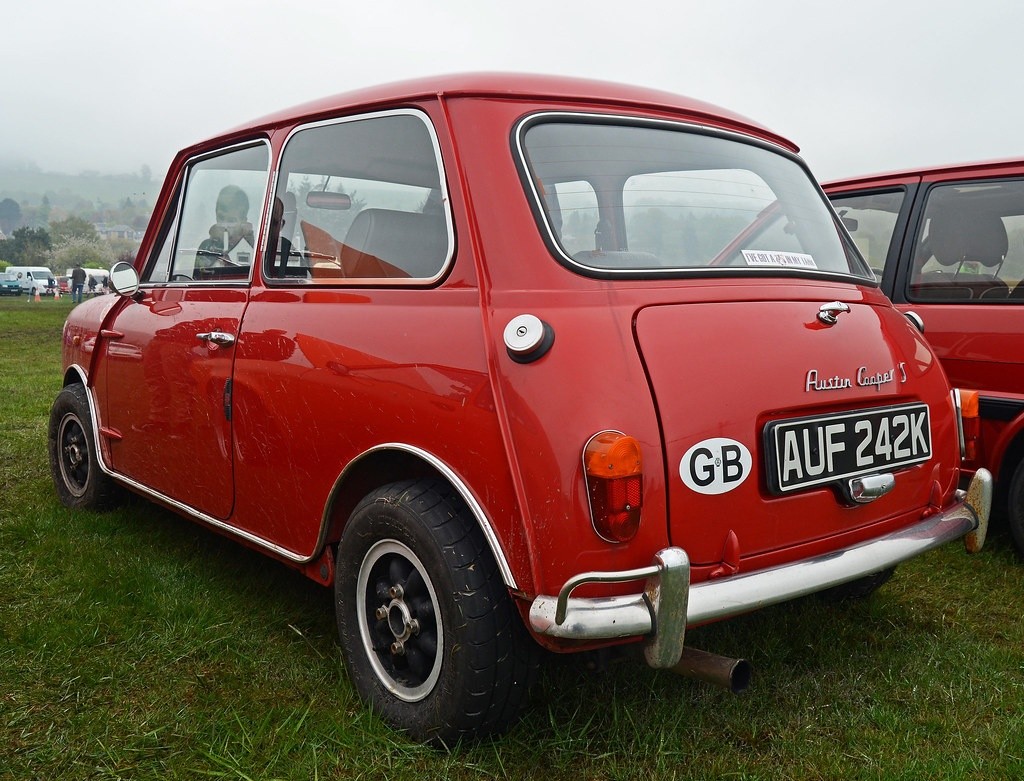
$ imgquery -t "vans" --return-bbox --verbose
[5,267,58,296]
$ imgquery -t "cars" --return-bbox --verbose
[0,273,24,297]
[48,72,993,759]
[709,156,1024,561]
[54,275,74,294]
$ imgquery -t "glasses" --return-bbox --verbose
[214,214,247,223]
[271,216,285,228]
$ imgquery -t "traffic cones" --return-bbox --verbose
[54,287,59,300]
[34,286,41,301]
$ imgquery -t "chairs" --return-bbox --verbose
[571,250,663,268]
[914,207,1010,299]
[339,208,447,276]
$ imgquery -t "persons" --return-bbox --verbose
[69,263,86,304]
[86,274,97,298]
[101,275,109,294]
[193,186,307,278]
[46,276,55,298]
